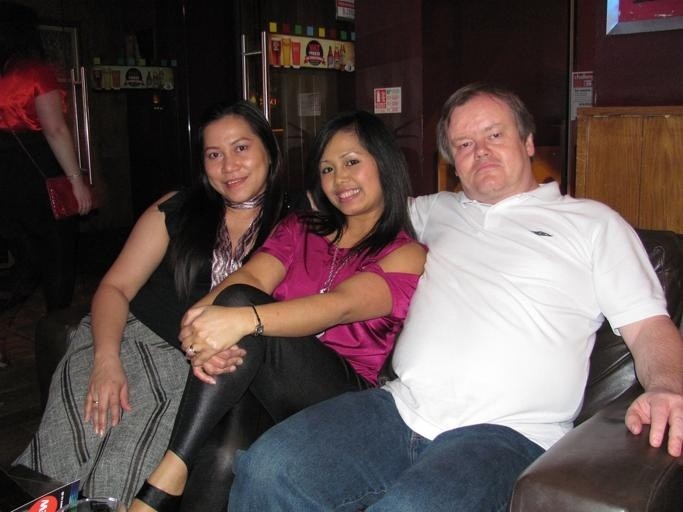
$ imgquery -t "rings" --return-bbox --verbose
[90,401,98,405]
[187,346,197,353]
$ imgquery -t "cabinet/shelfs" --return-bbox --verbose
[574,105,683,235]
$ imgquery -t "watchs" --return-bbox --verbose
[250,303,264,337]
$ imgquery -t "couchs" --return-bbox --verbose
[35,229,683,512]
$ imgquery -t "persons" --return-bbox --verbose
[227,83,683,512]
[13,100,302,508]
[129,113,426,512]
[0,14,92,368]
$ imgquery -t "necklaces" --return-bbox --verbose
[224,190,268,210]
[319,242,356,294]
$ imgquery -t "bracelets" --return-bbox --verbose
[65,170,82,183]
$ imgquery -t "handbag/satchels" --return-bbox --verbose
[45,173,109,221]
